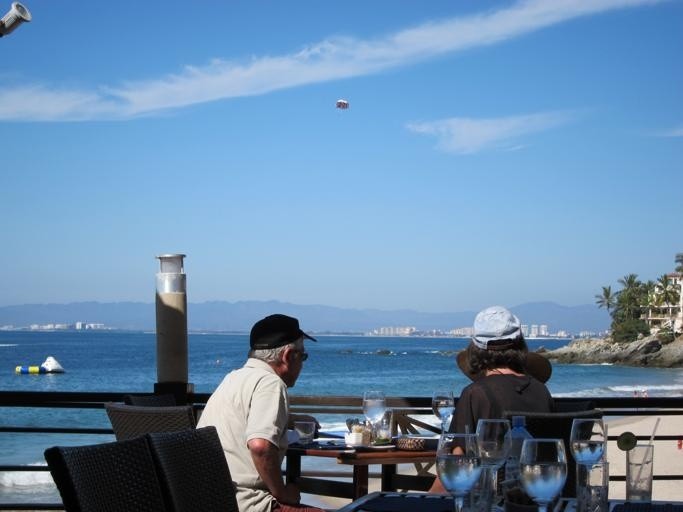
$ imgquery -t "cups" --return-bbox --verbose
[294,422,315,448]
[625,444,654,502]
[345,432,363,448]
[576,461,609,512]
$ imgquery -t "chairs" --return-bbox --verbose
[503,397,603,511]
[43,394,240,511]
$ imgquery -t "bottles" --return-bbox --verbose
[502,415,536,498]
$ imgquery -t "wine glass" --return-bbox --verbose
[476,418,513,512]
[432,390,454,452]
[518,438,568,512]
[361,390,387,443]
[570,418,607,483]
[436,433,482,512]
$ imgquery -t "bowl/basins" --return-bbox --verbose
[393,436,427,453]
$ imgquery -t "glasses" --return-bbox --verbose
[300,353,308,360]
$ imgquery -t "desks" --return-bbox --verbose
[278,426,450,510]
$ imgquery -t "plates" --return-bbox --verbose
[313,437,345,446]
[352,445,397,452]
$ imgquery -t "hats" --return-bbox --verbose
[470,306,522,350]
[248,314,318,349]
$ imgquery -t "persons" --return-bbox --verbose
[426,303,556,512]
[191,313,328,511]
